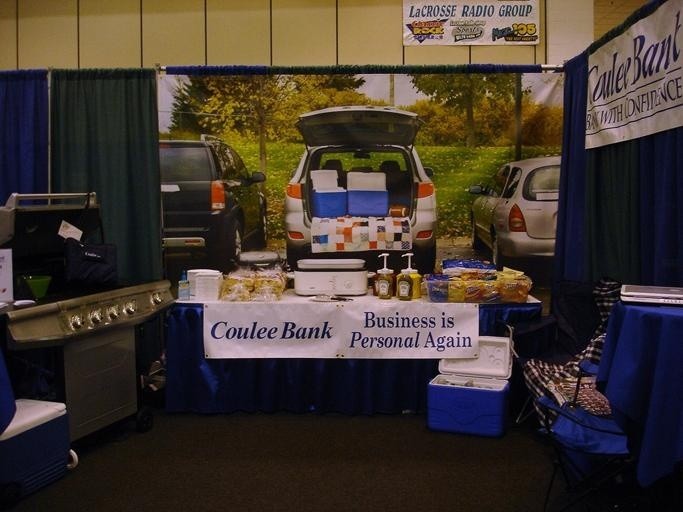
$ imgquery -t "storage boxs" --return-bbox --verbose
[423,331,518,439]
[307,169,347,217]
[1,395,73,502]
[346,171,389,217]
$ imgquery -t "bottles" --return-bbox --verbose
[178,271,189,299]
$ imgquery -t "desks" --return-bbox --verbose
[163,294,543,418]
[593,299,680,511]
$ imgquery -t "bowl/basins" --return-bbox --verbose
[24,275,51,298]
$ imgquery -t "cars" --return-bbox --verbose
[465,155,561,272]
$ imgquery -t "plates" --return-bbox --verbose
[309,296,338,302]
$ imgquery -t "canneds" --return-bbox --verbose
[373,268,421,300]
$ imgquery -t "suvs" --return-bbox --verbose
[157,133,269,272]
[279,105,440,279]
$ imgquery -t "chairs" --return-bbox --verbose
[534,358,639,511]
